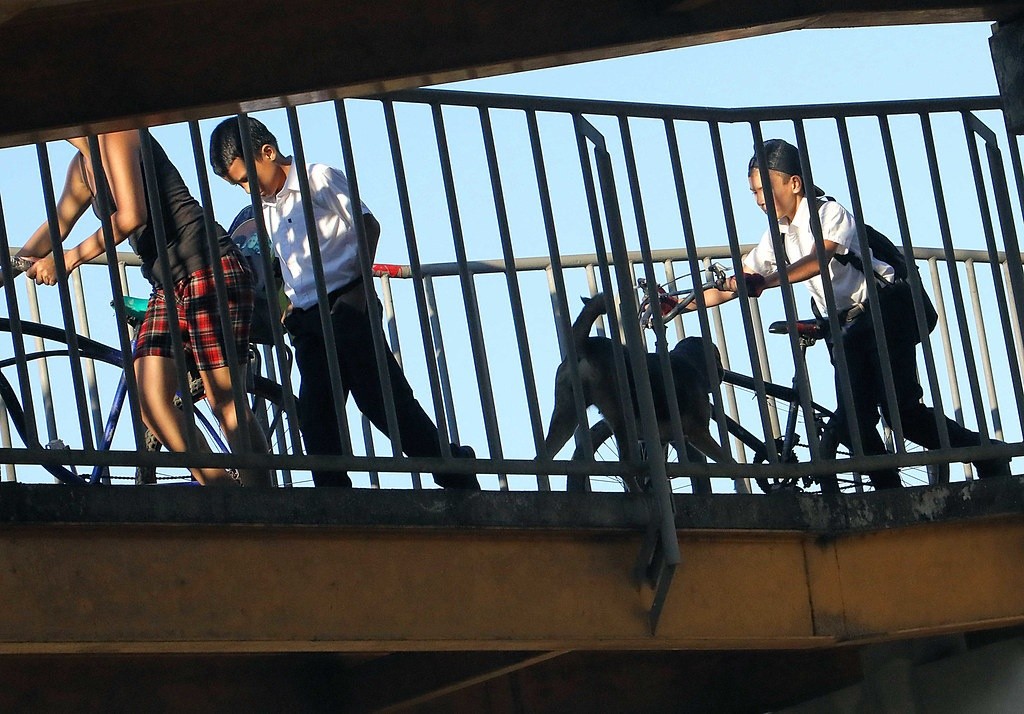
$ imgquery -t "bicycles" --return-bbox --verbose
[568,263,952,494]
[0,254,300,487]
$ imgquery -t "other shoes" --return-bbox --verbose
[970,438,1014,479]
[443,445,481,491]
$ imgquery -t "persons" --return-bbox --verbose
[210,117,482,491]
[0,126,271,487]
[640,140,1014,491]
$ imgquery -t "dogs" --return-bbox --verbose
[533,293,749,494]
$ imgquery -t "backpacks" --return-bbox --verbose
[808,194,938,346]
[228,207,290,346]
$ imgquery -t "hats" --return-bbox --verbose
[748,140,826,197]
